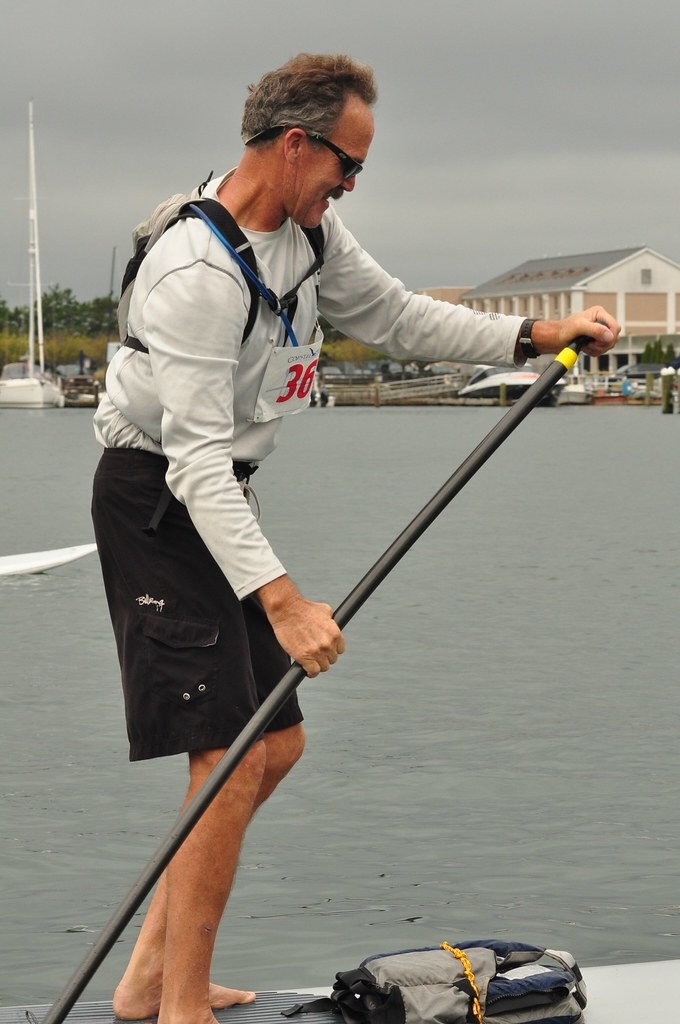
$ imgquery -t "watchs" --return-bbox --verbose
[519,318,541,359]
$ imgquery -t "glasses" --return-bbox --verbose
[243,126,364,178]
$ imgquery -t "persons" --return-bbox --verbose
[89,53,621,1022]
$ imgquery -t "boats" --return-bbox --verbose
[0,959,680,1024]
[457,362,567,408]
[1,543,99,576]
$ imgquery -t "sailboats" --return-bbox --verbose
[2,97,66,411]
